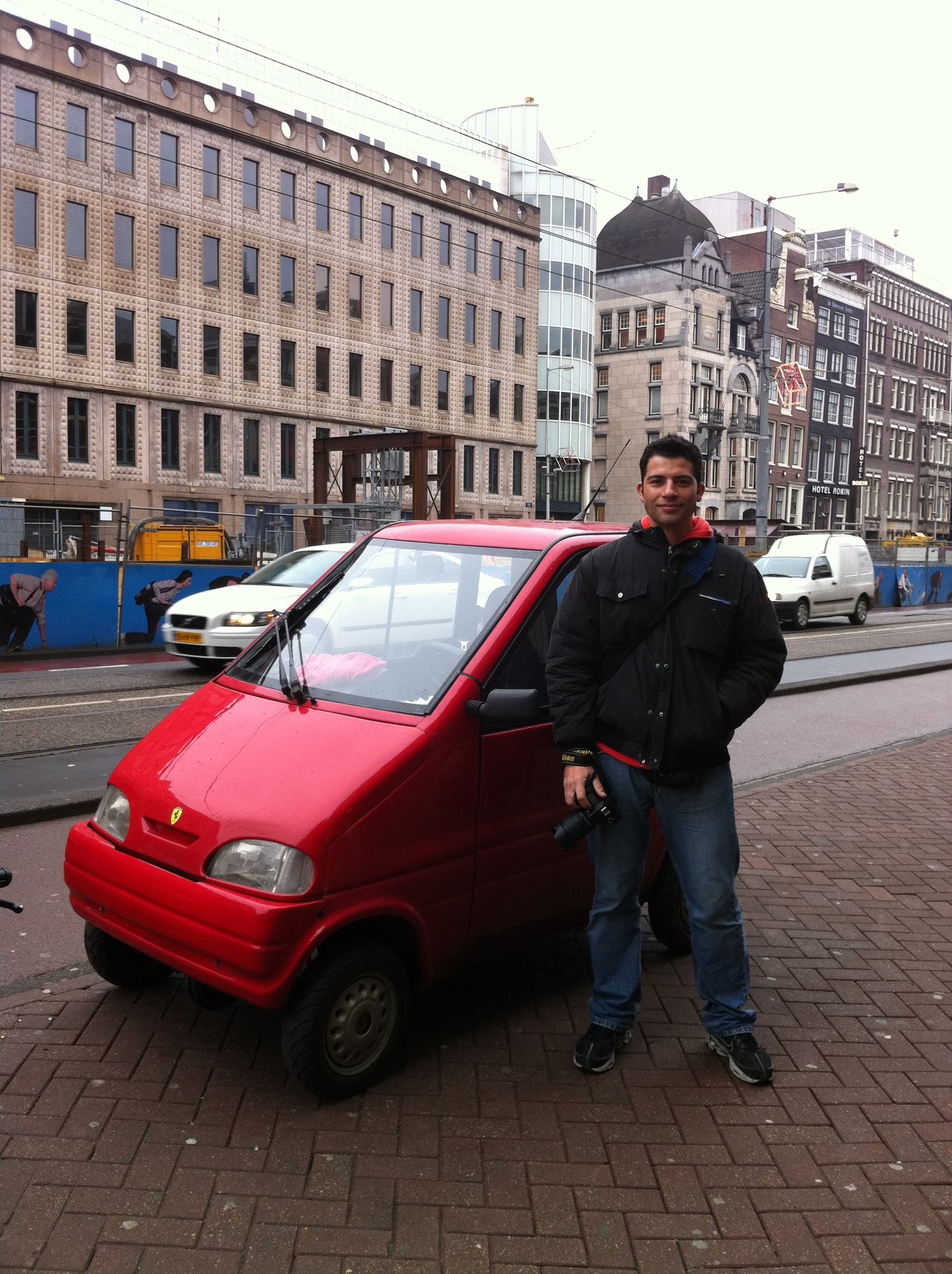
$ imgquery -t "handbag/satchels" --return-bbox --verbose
[0,584,20,611]
[134,580,156,606]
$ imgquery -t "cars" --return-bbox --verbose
[62,517,742,1103]
[159,542,509,671]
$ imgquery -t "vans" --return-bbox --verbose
[753,534,875,631]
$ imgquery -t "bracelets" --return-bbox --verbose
[42,640,46,642]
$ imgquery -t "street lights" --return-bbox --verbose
[751,181,862,552]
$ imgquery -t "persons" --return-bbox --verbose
[120,570,194,644]
[0,569,59,653]
[874,571,884,590]
[925,570,944,603]
[898,568,914,606]
[209,572,251,590]
[544,432,789,1082]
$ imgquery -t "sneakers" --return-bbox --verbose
[704,1028,774,1084]
[575,1023,632,1074]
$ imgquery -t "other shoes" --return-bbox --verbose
[120,632,126,646]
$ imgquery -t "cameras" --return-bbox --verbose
[552,785,620,847]
